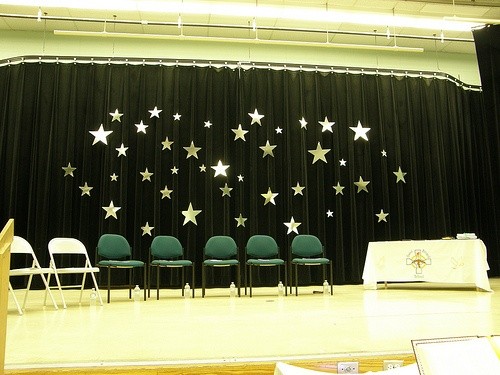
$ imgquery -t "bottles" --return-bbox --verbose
[230,282,235,297]
[134,285,140,302]
[323,280,329,296]
[185,283,191,300]
[90,288,97,306]
[278,281,283,297]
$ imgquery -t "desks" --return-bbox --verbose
[361,239,493,292]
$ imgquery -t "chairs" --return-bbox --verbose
[148,236,194,300]
[245,235,287,297]
[290,235,333,296]
[43,238,103,308]
[9,236,58,314]
[95,235,146,303]
[202,236,240,298]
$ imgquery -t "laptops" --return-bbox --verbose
[411,334,500,375]
